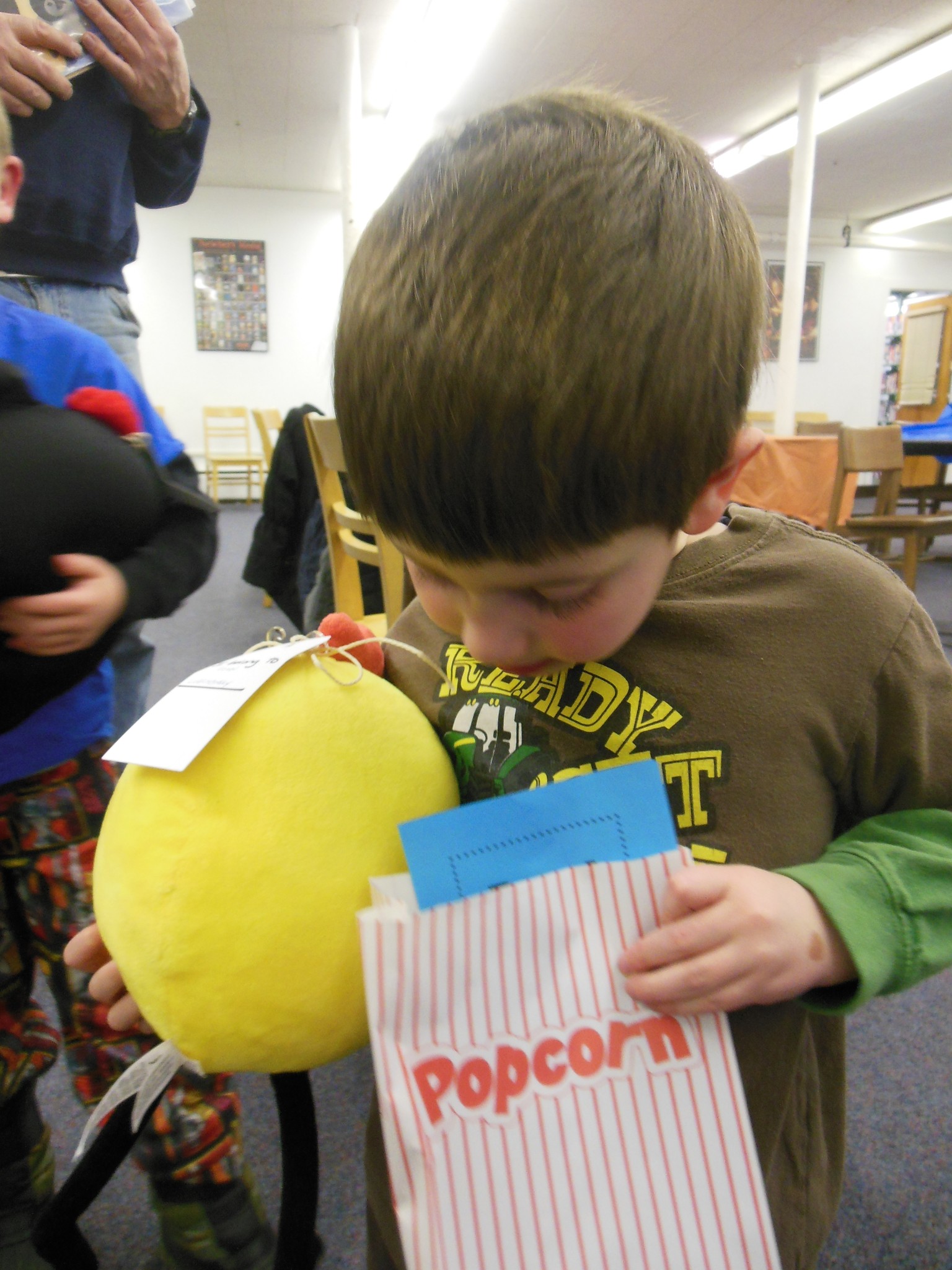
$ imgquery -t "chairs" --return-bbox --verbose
[203,406,265,505]
[745,410,952,592]
[252,407,286,467]
[301,411,407,641]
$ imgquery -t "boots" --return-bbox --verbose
[0,1119,54,1270]
[154,1172,275,1269]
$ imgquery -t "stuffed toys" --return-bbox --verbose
[91,613,459,1073]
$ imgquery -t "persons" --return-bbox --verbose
[0,0,273,1270]
[63,87,952,1270]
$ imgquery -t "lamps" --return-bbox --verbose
[866,194,952,234]
[711,29,952,180]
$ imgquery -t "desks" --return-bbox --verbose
[732,434,860,525]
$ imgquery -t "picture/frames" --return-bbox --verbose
[192,237,269,351]
[761,259,824,362]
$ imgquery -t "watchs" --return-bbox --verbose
[153,95,198,135]
